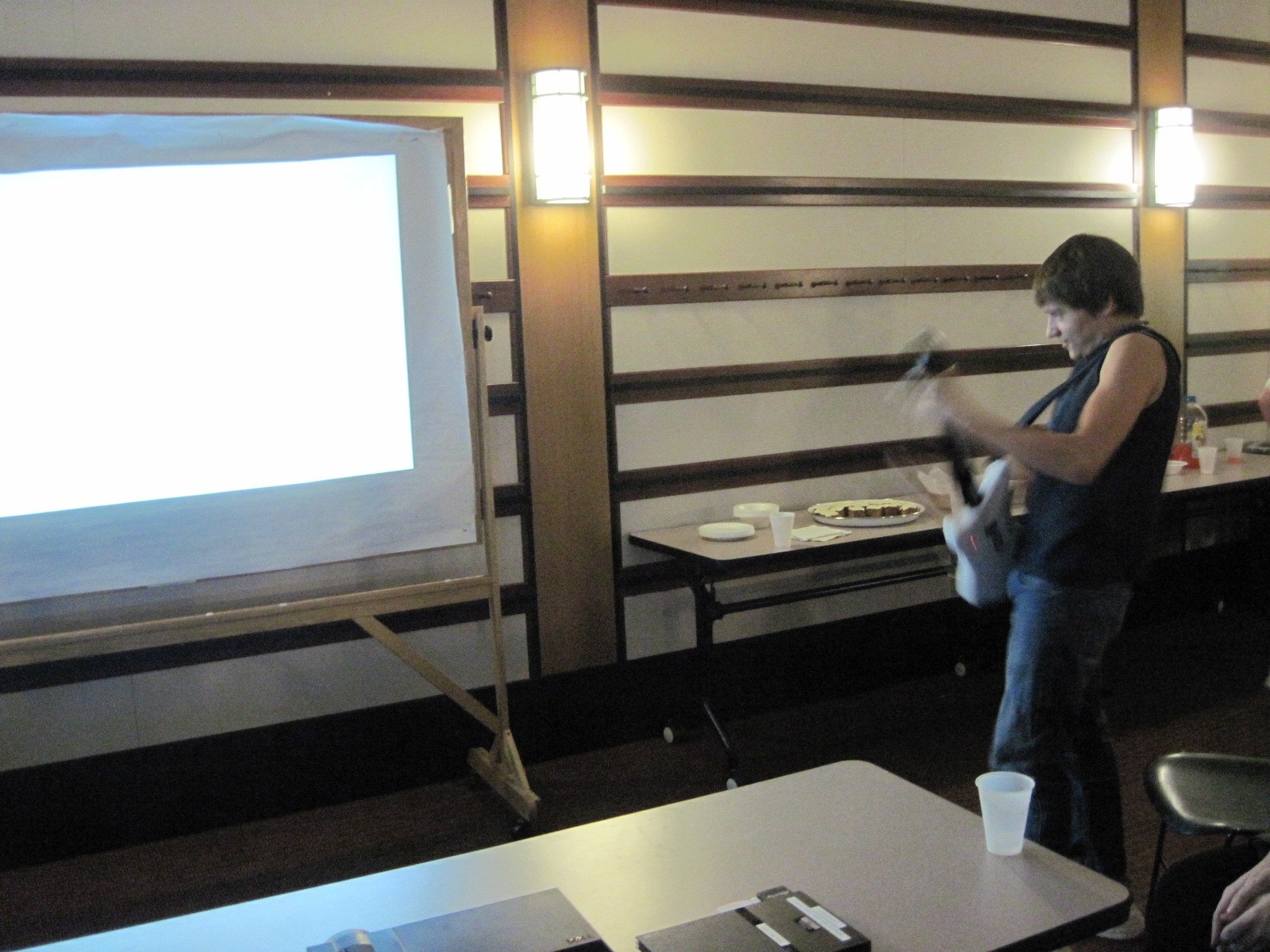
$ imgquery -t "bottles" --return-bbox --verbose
[1167,395,1209,469]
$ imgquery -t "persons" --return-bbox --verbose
[924,236,1180,888]
[1210,851,1270,952]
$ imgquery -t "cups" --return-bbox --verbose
[1226,438,1243,465]
[1199,447,1218,475]
[975,770,1035,856]
[770,511,796,549]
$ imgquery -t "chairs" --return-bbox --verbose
[1144,753,1269,899]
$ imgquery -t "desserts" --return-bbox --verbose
[811,499,920,518]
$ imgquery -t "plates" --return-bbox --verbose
[807,501,926,527]
[700,523,756,539]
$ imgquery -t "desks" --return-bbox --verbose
[10,442,1270,952]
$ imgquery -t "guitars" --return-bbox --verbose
[895,325,1024,609]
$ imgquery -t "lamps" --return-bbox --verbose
[529,68,595,204]
[1153,107,1197,205]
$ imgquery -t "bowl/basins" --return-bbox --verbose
[735,502,778,528]
[1166,460,1189,475]
